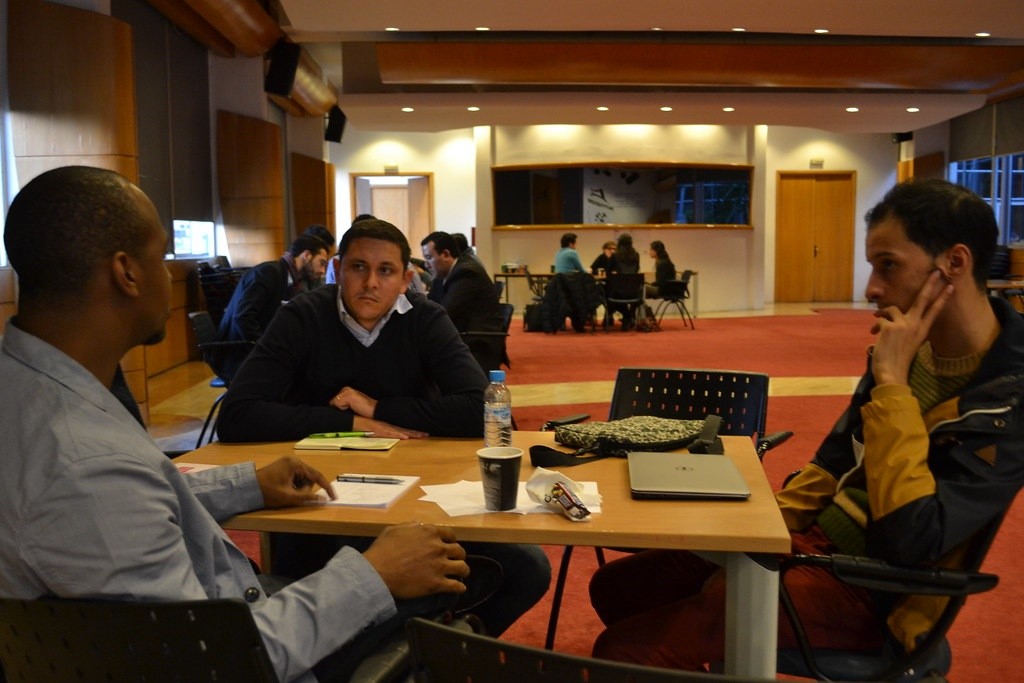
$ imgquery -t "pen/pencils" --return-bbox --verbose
[336,476,405,484]
[309,431,375,438]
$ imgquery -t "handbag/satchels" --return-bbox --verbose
[525,303,564,332]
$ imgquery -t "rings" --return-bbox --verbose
[336,395,340,400]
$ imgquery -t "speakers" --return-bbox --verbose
[892,131,913,143]
[325,106,346,143]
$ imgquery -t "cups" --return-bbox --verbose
[598,268,604,276]
[476,447,524,511]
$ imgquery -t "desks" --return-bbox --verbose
[171,436,792,678]
[988,279,1024,297]
[494,273,645,303]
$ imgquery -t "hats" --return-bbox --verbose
[604,242,617,249]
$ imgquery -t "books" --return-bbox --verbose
[294,437,400,451]
[312,473,420,509]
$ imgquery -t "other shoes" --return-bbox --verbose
[601,319,615,326]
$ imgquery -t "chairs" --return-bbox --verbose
[539,368,793,650]
[187,255,257,450]
[521,264,698,332]
[462,280,519,432]
[0,595,488,683]
[710,500,1009,683]
[404,616,758,682]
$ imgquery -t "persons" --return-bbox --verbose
[554,233,593,274]
[214,224,336,373]
[421,231,499,332]
[605,233,640,330]
[591,241,616,326]
[326,214,377,284]
[588,176,1024,674]
[216,219,552,636]
[642,241,676,298]
[0,166,467,683]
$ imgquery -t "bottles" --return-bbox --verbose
[484,370,512,448]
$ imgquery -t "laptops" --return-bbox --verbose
[626,452,751,498]
